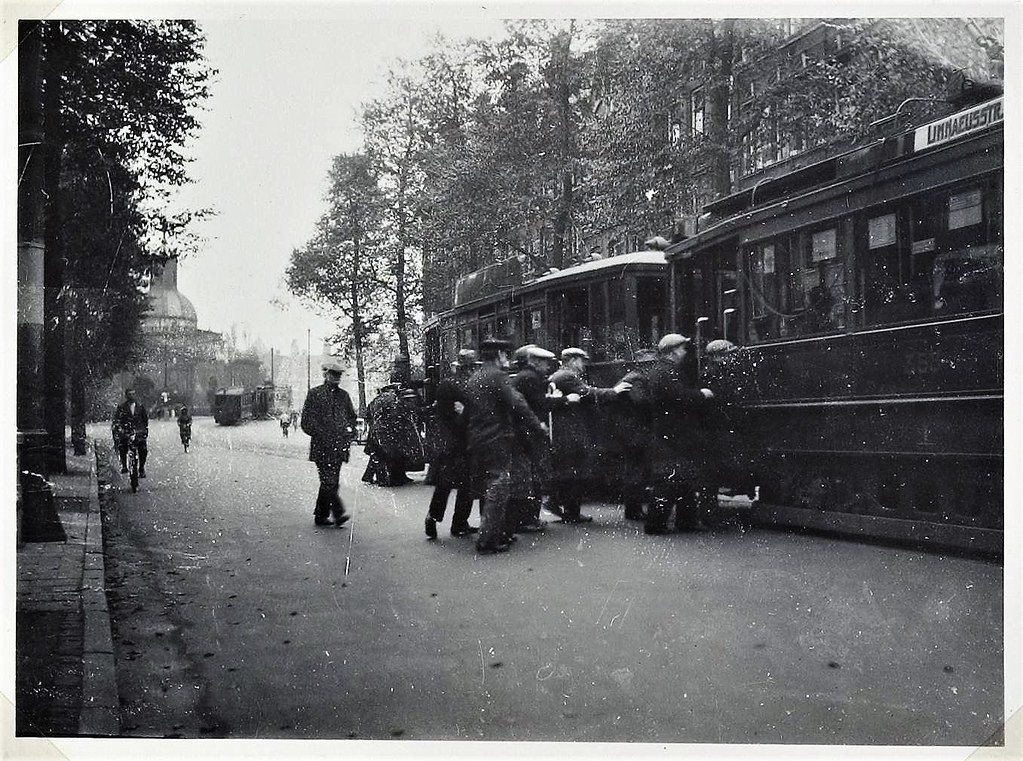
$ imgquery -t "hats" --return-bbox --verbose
[705,339,739,355]
[658,333,691,353]
[320,363,346,373]
[375,380,420,400]
[634,349,659,363]
[435,333,591,374]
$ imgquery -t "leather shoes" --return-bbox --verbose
[314,516,334,526]
[425,517,439,539]
[333,515,349,526]
[478,475,734,555]
[451,522,479,537]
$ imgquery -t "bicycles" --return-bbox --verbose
[179,423,193,451]
[282,423,290,436]
[117,428,148,491]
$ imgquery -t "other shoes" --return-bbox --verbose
[356,469,418,490]
[139,467,146,478]
[121,468,128,473]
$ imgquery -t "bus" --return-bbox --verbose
[249,386,292,418]
[666,68,1005,561]
[418,236,669,508]
[214,386,253,426]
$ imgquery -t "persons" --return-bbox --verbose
[279,409,299,430]
[299,363,359,529]
[116,389,149,478]
[360,336,741,556]
[177,406,192,446]
